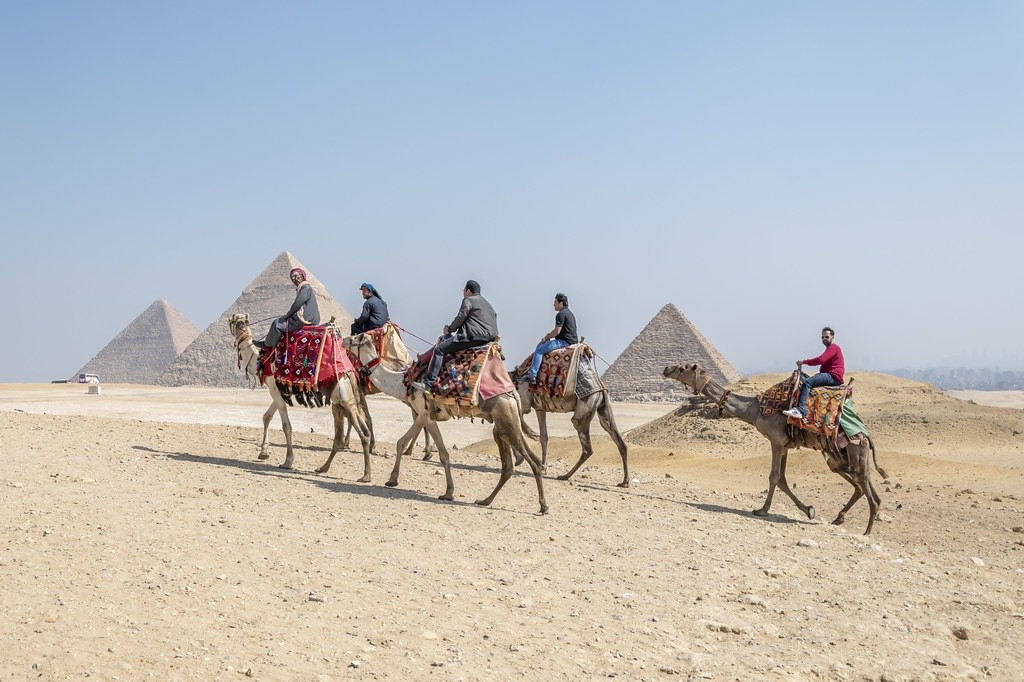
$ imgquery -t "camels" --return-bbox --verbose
[343,332,548,516]
[229,313,433,484]
[507,336,630,488]
[663,361,889,536]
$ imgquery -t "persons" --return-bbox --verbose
[349,283,389,337]
[408,280,498,392]
[251,268,320,350]
[520,292,578,386]
[781,326,844,419]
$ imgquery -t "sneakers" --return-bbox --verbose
[782,407,802,418]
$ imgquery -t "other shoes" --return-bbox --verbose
[252,340,272,351]
[411,381,431,396]
[520,376,536,384]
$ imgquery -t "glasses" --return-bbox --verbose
[821,335,829,340]
